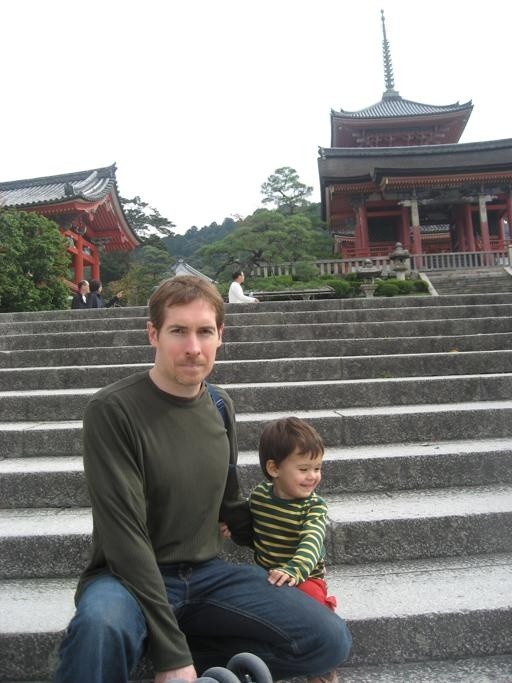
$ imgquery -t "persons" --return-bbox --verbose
[218,415,338,616]
[228,269,260,302]
[50,273,354,682]
[89,278,124,308]
[72,279,95,309]
